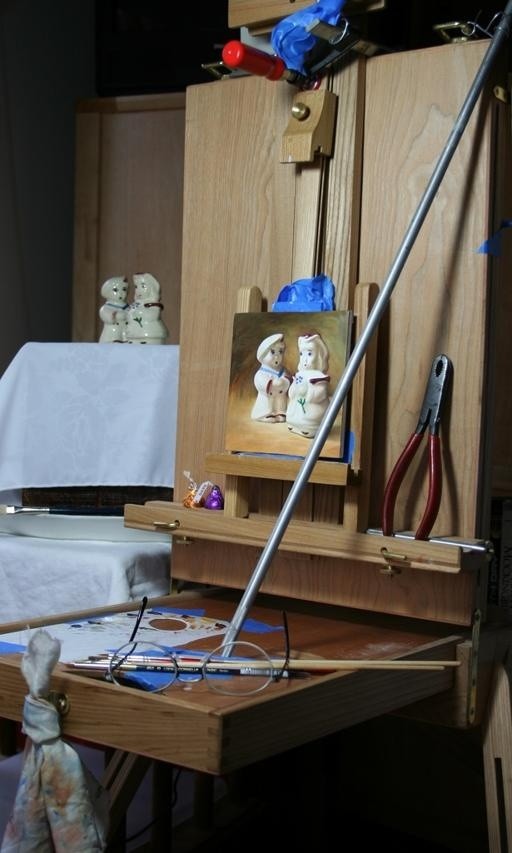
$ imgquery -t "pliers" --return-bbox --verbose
[381,354,453,541]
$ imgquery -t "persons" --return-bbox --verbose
[98,275,130,343]
[125,272,168,344]
[250,333,293,422]
[286,329,330,437]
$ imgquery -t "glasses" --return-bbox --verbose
[109,596,291,695]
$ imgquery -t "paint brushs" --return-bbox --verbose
[65,654,463,679]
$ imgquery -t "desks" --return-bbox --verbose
[0,590,470,851]
